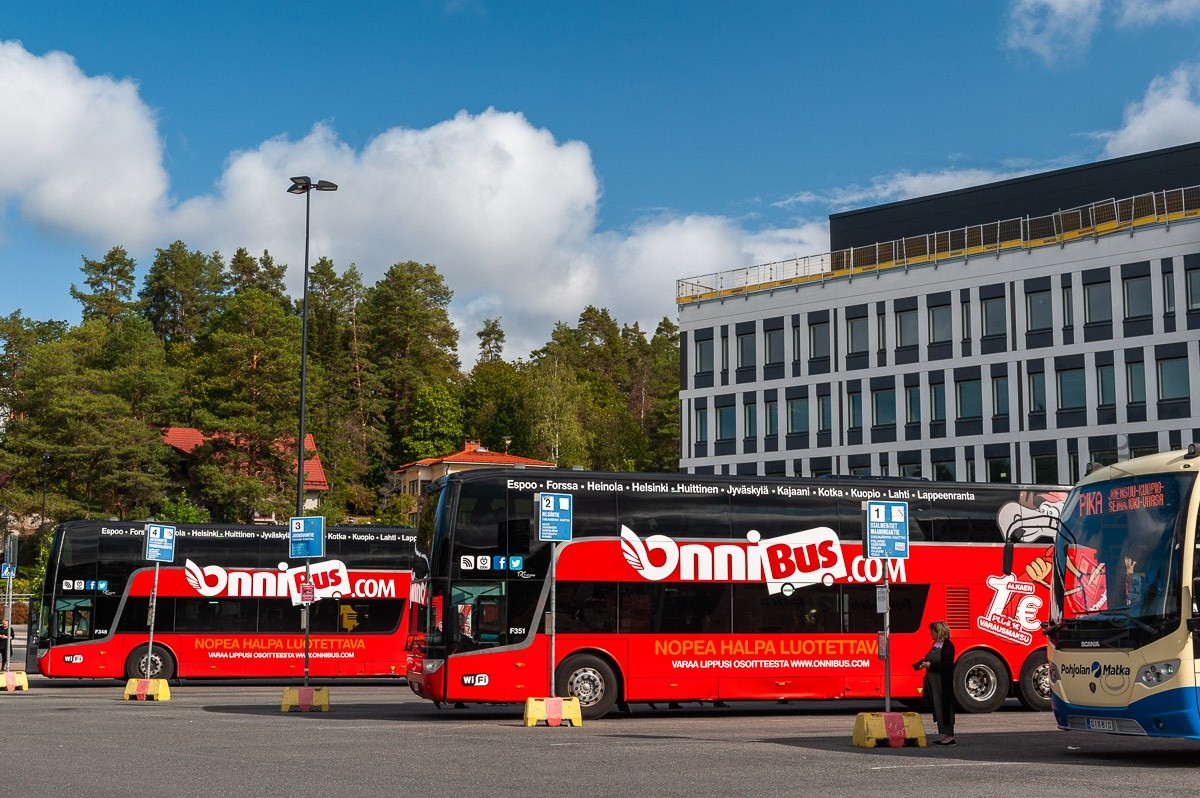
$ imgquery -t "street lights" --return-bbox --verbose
[287,175,339,518]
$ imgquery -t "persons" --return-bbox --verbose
[0,619,14,671]
[69,610,89,638]
[916,621,956,746]
[435,613,470,709]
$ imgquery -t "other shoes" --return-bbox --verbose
[933,739,956,746]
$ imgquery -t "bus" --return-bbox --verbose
[33,518,506,688]
[404,466,1138,727]
[999,444,1200,740]
